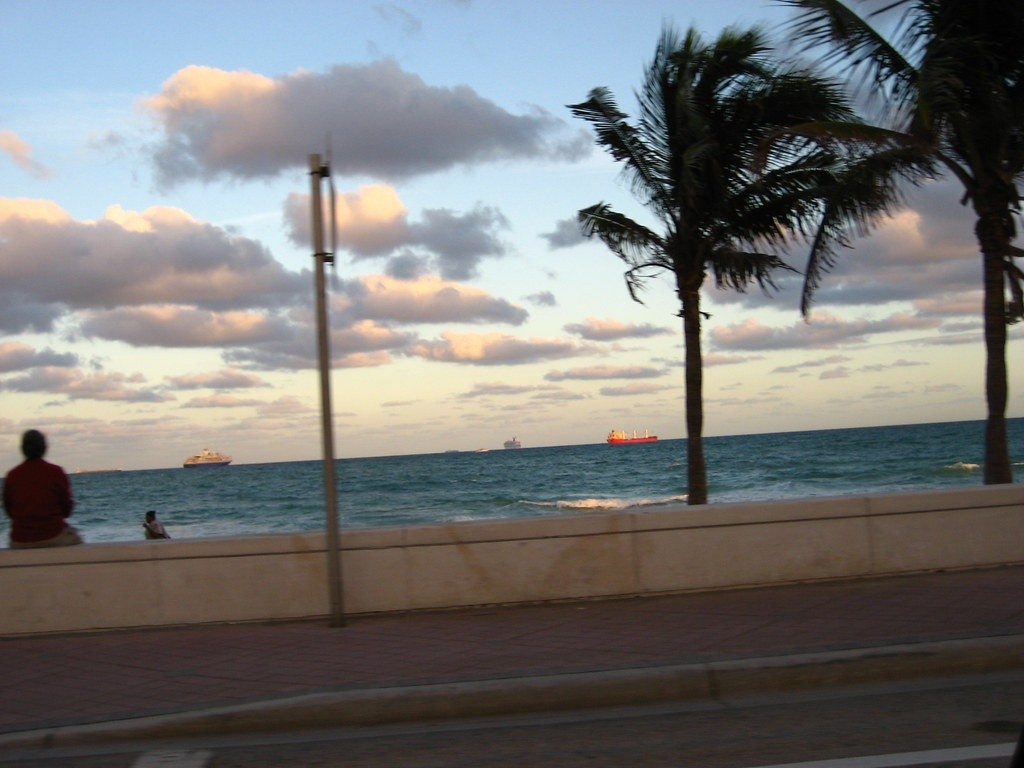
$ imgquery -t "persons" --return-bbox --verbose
[142,509,172,542]
[0,429,83,550]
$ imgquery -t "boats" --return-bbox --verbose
[183,448,233,468]
[606,429,658,446]
